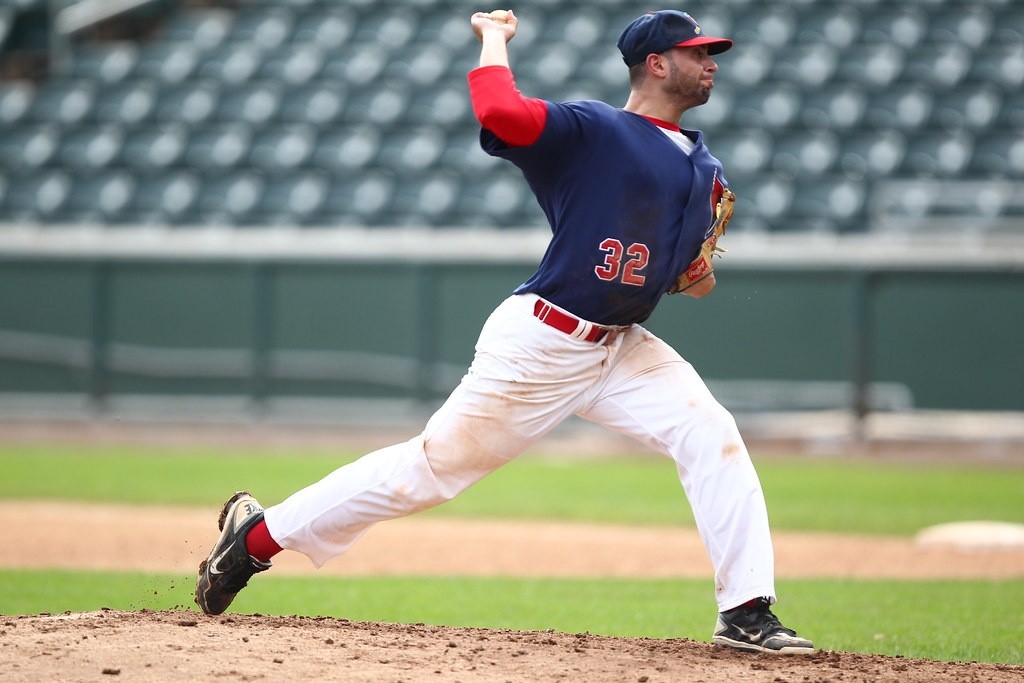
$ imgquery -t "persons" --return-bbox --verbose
[196,9,812,653]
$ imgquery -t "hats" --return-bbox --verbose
[616,10,734,69]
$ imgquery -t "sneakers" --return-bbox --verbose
[713,596,816,656]
[191,490,274,615]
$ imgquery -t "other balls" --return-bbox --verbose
[489,9,509,24]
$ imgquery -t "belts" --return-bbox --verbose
[533,300,625,347]
